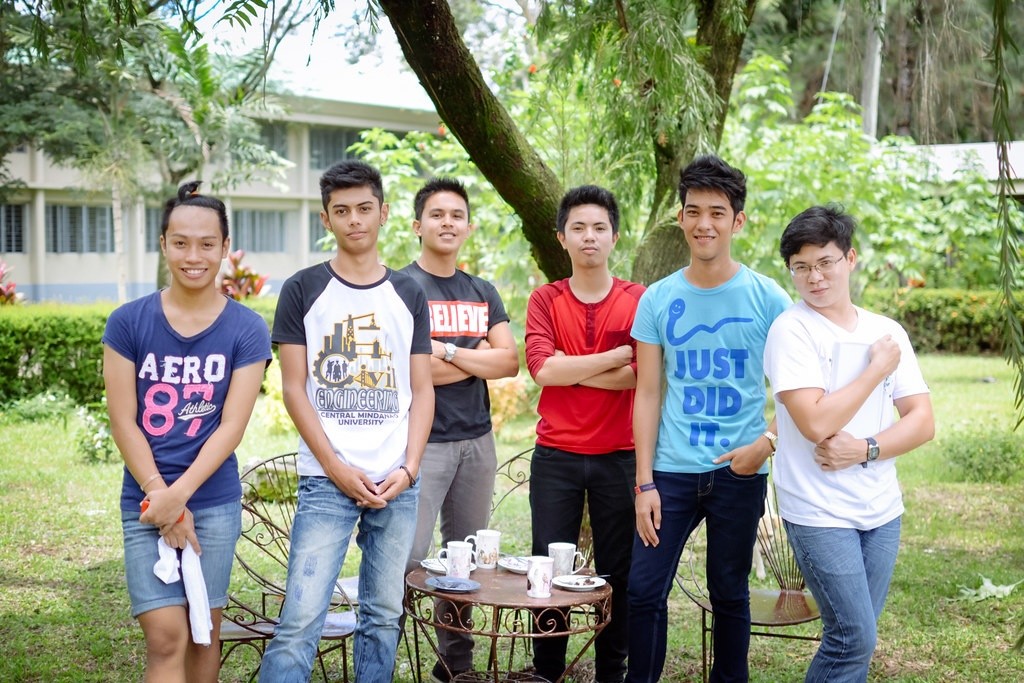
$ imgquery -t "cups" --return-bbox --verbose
[527,556,554,598]
[437,541,474,581]
[548,542,587,577]
[463,529,501,570]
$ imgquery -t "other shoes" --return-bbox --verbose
[431,657,478,683]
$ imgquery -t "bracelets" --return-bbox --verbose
[400,465,416,488]
[634,482,656,494]
[571,383,579,386]
[140,474,161,491]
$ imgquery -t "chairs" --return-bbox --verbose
[216,505,358,683]
[676,451,823,683]
[238,451,416,683]
[489,445,595,683]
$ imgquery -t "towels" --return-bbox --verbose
[152,535,214,649]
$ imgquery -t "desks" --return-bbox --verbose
[402,565,614,683]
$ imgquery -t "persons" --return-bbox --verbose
[258,163,435,683]
[524,184,664,683]
[623,154,795,683]
[103,181,272,683]
[397,176,519,683]
[763,206,935,683]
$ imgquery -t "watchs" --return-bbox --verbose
[861,437,880,468]
[444,342,457,362]
[764,430,778,456]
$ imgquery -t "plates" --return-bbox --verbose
[420,558,477,575]
[551,574,606,591]
[425,576,481,593]
[498,556,531,574]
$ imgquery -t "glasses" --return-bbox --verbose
[786,248,851,280]
